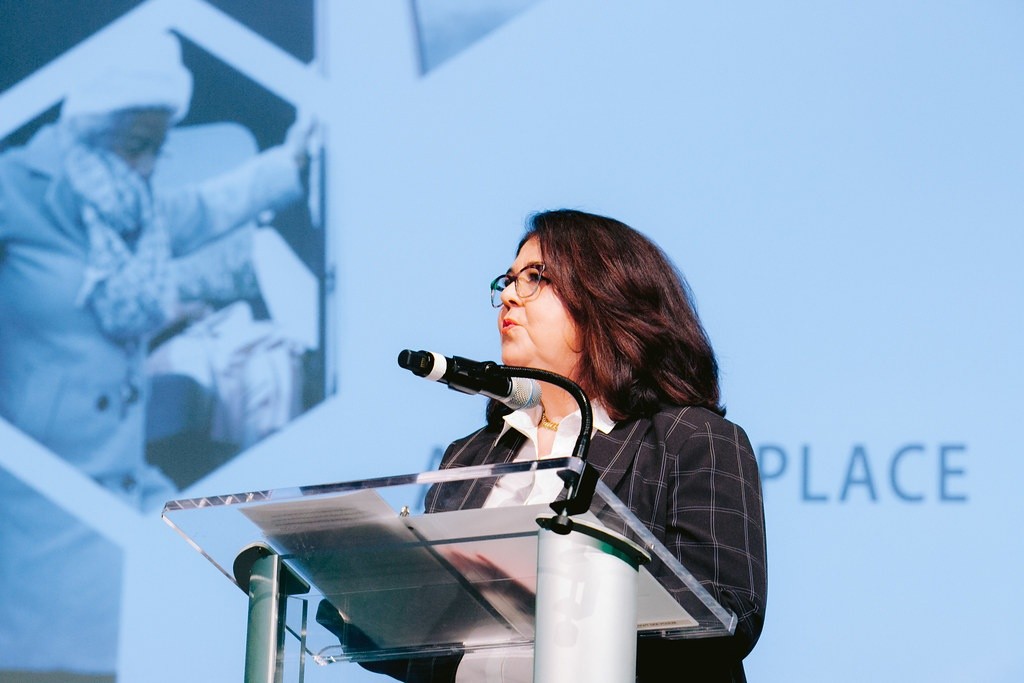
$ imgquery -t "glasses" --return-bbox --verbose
[491,264,546,308]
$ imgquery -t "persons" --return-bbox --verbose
[1,32,332,504]
[313,209,769,683]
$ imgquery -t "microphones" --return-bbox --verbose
[398,349,543,410]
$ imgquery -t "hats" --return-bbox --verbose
[59,33,192,128]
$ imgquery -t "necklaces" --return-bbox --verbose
[538,414,559,435]
